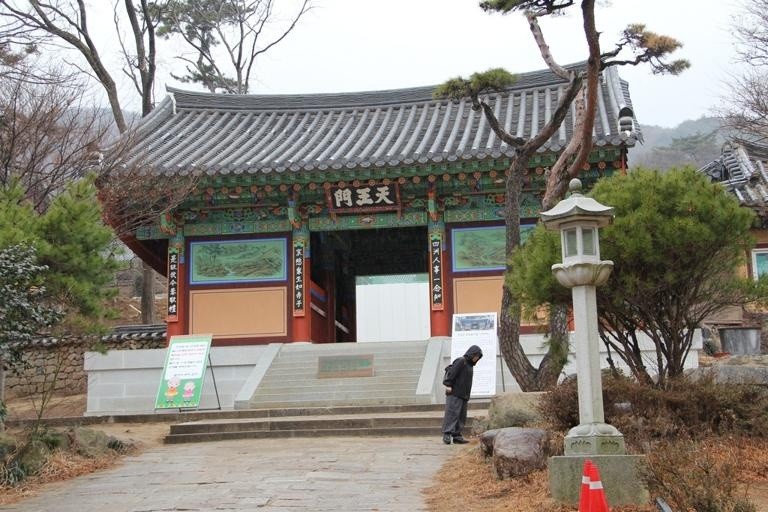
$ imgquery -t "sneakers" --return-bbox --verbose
[443,433,451,444]
[453,434,470,444]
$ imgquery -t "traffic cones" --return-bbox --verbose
[578,459,609,512]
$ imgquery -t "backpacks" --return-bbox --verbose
[443,364,453,385]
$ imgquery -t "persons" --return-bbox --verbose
[442,345,483,444]
